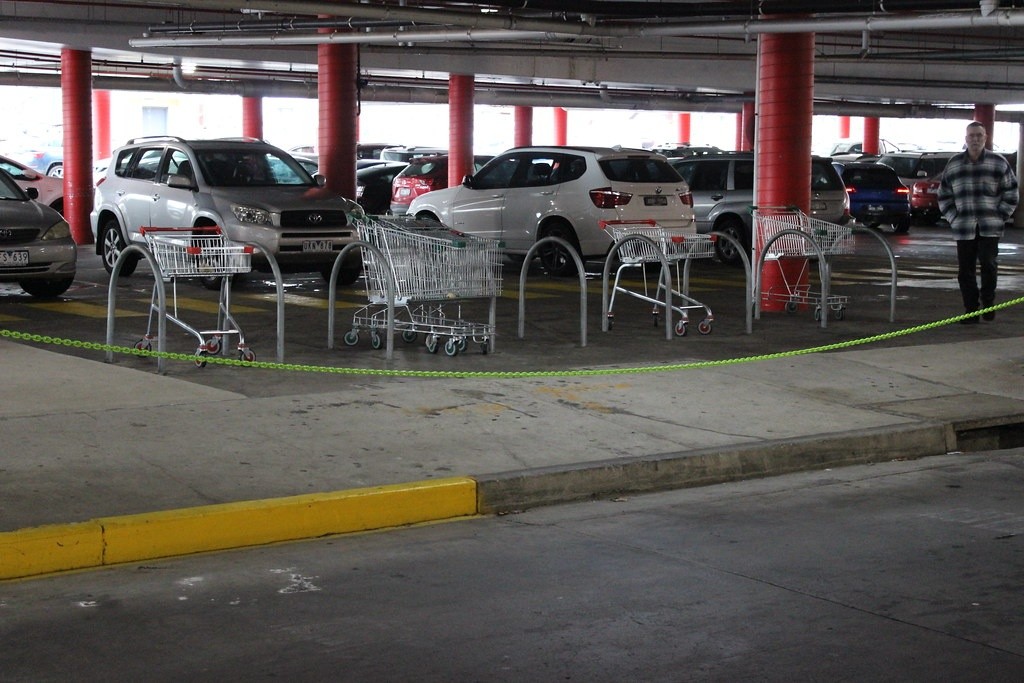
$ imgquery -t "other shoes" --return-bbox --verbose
[959,308,980,324]
[982,301,996,321]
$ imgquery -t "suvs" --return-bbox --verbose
[89,136,363,291]
[390,138,1017,268]
[403,147,696,280]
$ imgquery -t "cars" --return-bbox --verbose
[0,144,448,222]
[0,168,75,300]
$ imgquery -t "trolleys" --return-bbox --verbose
[746,204,853,321]
[134,226,256,369]
[598,219,716,336]
[344,210,505,358]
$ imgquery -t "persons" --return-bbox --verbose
[936,122,1020,325]
